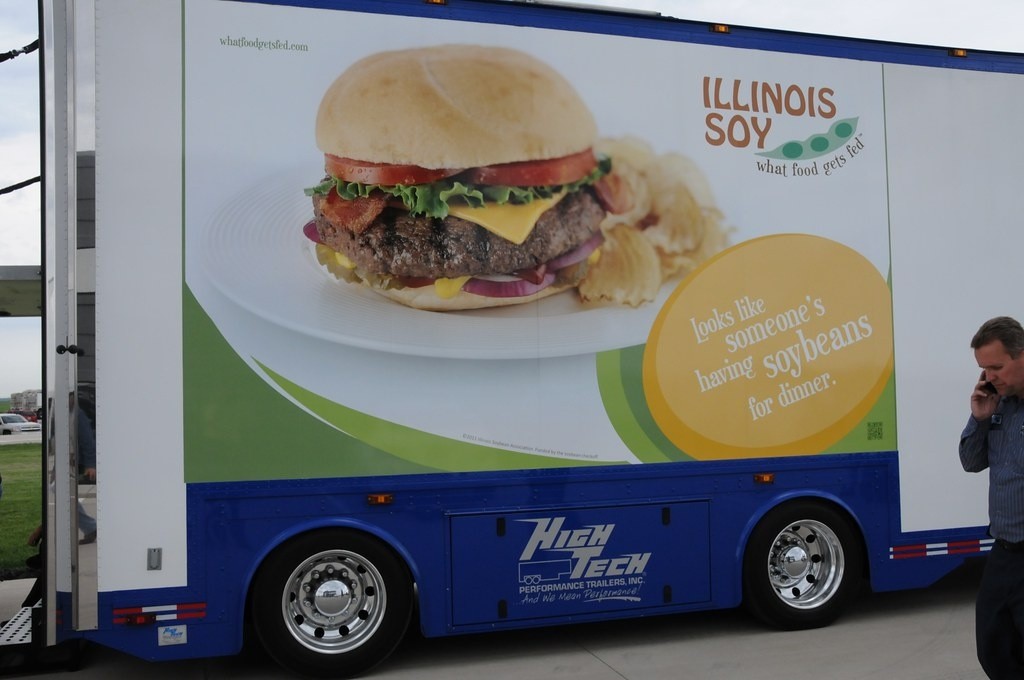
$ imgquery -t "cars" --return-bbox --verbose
[8,410,38,423]
[0,413,42,435]
[35,407,43,419]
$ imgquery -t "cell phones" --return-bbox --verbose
[983,375,997,393]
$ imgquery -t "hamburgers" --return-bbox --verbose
[301,42,629,311]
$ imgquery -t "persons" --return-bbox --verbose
[959,316,1024,680]
[28,525,42,546]
[77,405,97,545]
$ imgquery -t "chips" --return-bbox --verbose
[576,135,728,306]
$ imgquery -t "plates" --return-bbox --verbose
[202,211,723,360]
